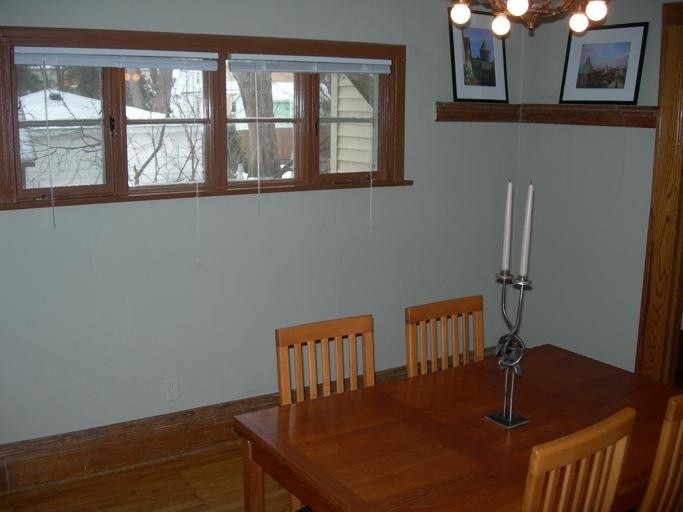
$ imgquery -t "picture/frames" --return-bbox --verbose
[553,20,651,105]
[445,6,512,105]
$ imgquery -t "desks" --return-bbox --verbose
[231,341,682,512]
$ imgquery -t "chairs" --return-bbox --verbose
[638,393,682,510]
[399,291,487,379]
[268,311,378,511]
[520,403,638,510]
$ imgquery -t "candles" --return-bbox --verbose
[516,178,536,277]
[498,178,515,273]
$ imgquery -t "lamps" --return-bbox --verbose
[445,0,614,42]
